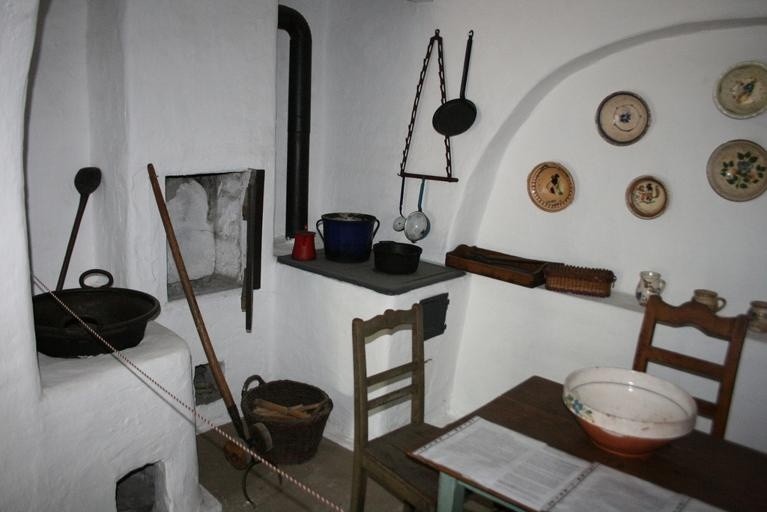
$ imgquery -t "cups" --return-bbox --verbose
[636,270,766,332]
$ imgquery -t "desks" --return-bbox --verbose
[405,373,766,508]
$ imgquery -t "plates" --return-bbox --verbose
[528,62,766,219]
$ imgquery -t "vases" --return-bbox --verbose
[291,212,379,263]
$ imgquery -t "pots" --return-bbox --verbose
[33,268,162,358]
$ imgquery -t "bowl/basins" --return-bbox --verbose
[372,241,422,276]
[562,365,698,459]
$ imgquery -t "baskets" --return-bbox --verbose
[241,374,333,465]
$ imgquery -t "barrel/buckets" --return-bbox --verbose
[316,213,380,264]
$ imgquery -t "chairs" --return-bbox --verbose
[629,295,750,441]
[350,302,469,511]
